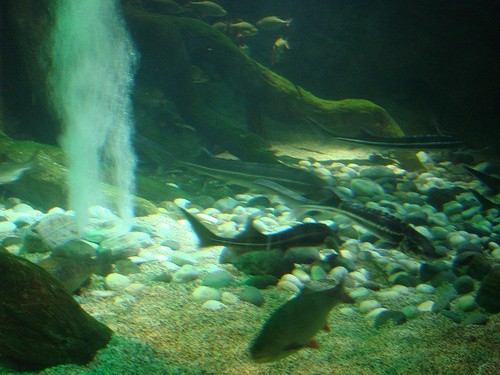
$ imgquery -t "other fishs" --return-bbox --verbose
[133,0,293,71]
[175,116,500,365]
[0,148,139,296]
